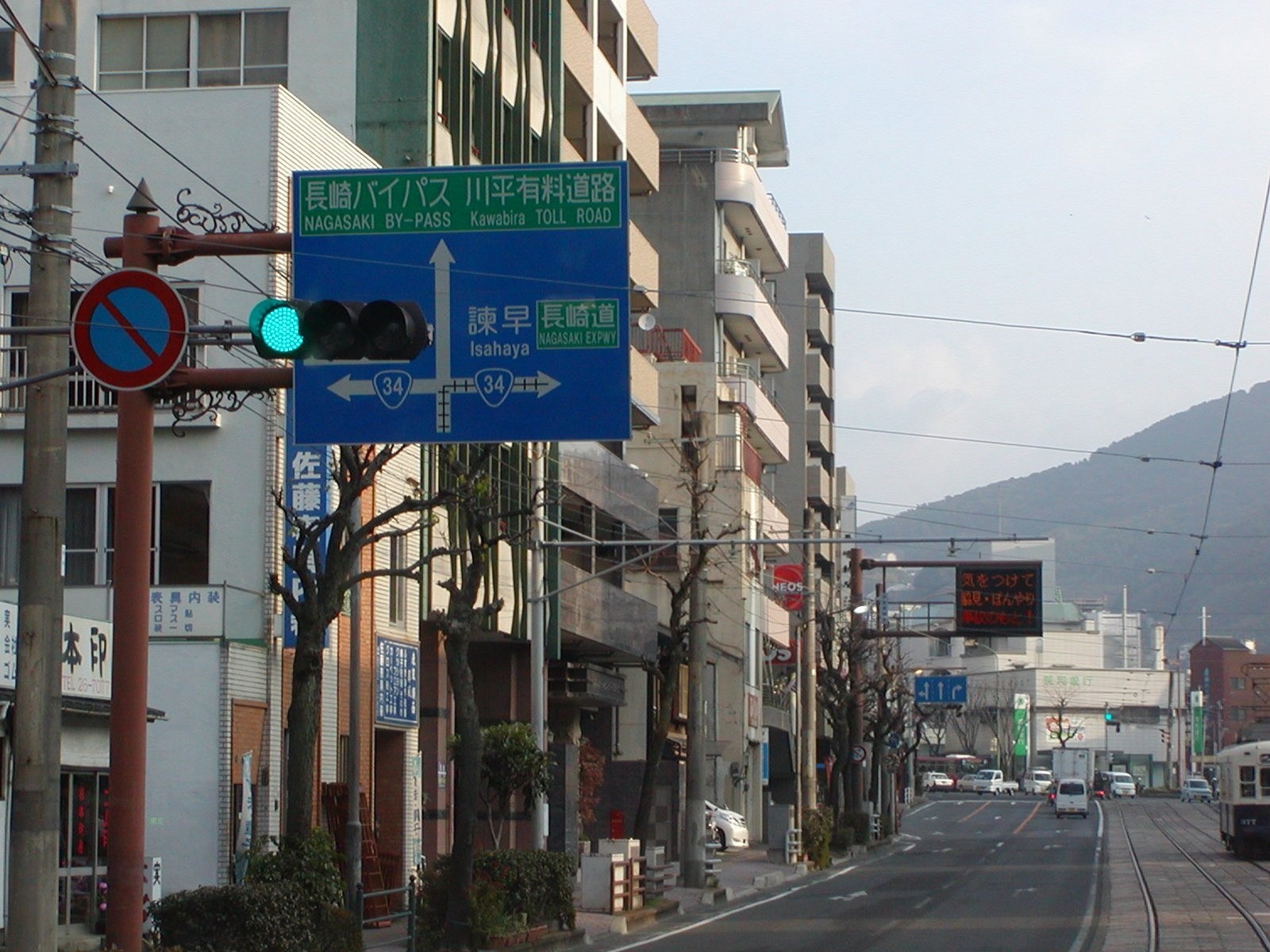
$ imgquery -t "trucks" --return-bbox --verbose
[1050,748,1096,786]
[973,769,1020,797]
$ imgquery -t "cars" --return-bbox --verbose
[956,774,978,793]
[1181,778,1213,804]
[704,800,750,852]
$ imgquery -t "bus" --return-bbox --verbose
[1215,737,1270,861]
[944,753,989,784]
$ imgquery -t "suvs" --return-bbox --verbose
[922,771,955,792]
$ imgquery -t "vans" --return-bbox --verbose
[1022,769,1054,796]
[1053,777,1089,819]
[1099,770,1137,799]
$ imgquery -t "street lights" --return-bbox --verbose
[961,638,1001,770]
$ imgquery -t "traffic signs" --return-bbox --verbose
[292,161,632,445]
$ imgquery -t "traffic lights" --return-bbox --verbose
[1106,713,1112,725]
[247,298,430,361]
